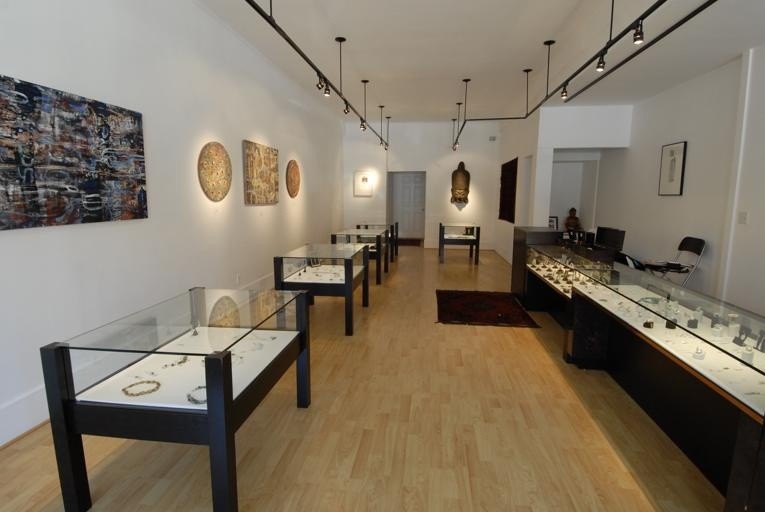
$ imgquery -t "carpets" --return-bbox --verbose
[435,288,540,331]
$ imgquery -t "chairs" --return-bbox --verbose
[645,235,707,289]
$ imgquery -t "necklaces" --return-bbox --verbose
[122,380,160,397]
[189,385,207,405]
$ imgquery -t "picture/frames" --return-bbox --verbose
[354,170,373,197]
[656,140,689,197]
[550,215,558,230]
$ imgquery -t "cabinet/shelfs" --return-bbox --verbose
[330,220,402,287]
[571,265,765,511]
[512,224,563,313]
[37,284,314,512]
[272,241,372,339]
[527,244,620,365]
[437,221,483,265]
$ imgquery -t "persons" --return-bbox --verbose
[565,207,584,231]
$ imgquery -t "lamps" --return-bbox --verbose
[315,74,390,152]
[560,16,646,99]
